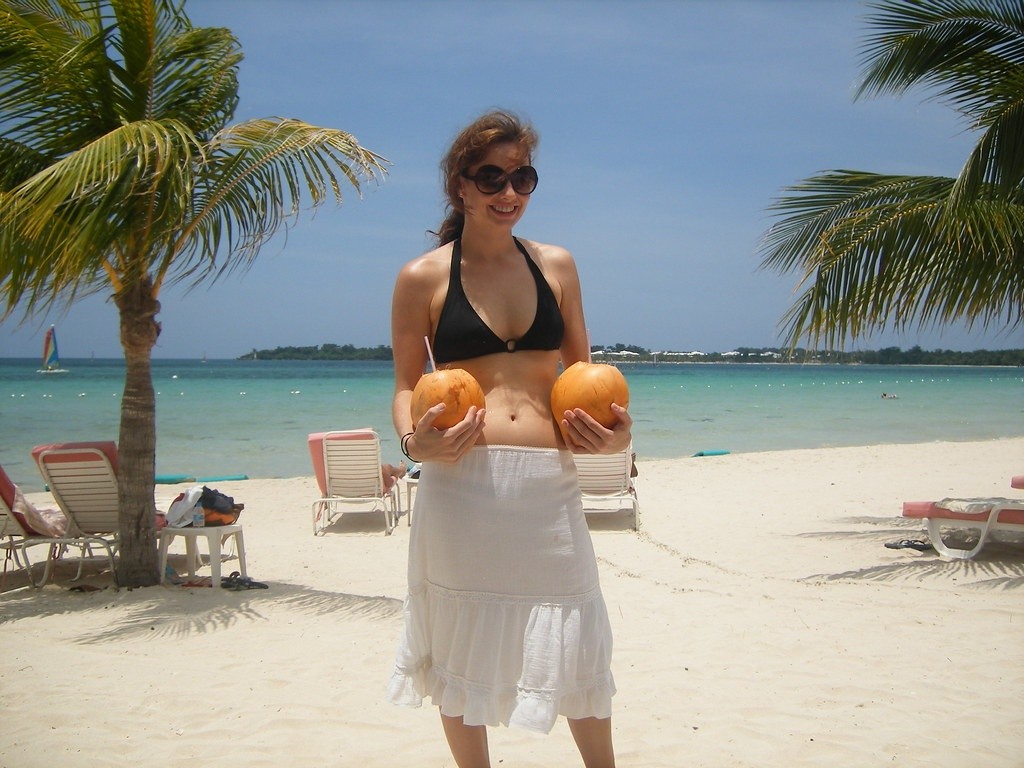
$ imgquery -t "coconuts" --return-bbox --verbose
[551,361,628,446]
[410,368,486,430]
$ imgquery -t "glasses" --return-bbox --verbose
[462,164,539,196]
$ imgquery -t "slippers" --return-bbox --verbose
[221,572,243,588]
[230,581,269,591]
[884,539,931,550]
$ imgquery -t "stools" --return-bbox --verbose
[158,524,246,589]
[402,475,419,527]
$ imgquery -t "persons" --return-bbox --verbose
[382,460,410,489]
[388,110,633,768]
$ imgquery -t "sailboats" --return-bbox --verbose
[35,325,70,374]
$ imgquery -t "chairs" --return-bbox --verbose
[573,439,640,532]
[308,427,400,535]
[903,497,1024,559]
[0,441,236,593]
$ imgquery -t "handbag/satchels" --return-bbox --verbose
[203,502,245,526]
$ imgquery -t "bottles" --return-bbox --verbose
[192,501,205,528]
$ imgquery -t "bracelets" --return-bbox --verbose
[400,432,421,463]
